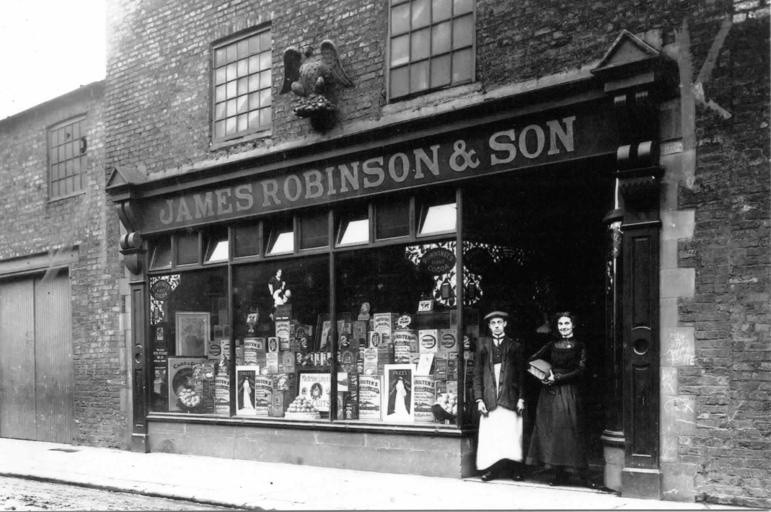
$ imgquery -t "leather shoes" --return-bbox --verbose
[515,473,523,480]
[481,470,497,480]
[549,479,560,486]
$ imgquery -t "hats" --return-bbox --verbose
[483,311,509,320]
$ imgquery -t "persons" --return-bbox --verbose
[267,269,283,296]
[473,310,527,481]
[525,312,598,490]
